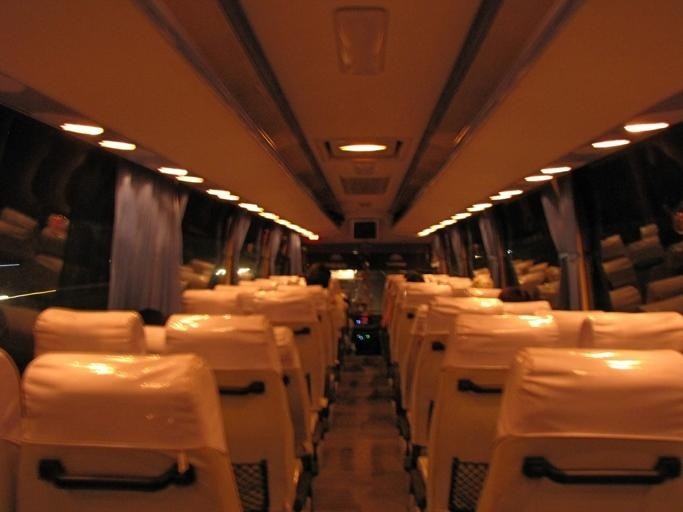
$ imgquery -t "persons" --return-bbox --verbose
[305,258,332,287]
[498,288,534,305]
[404,269,426,283]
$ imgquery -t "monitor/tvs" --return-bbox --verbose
[351,217,379,243]
